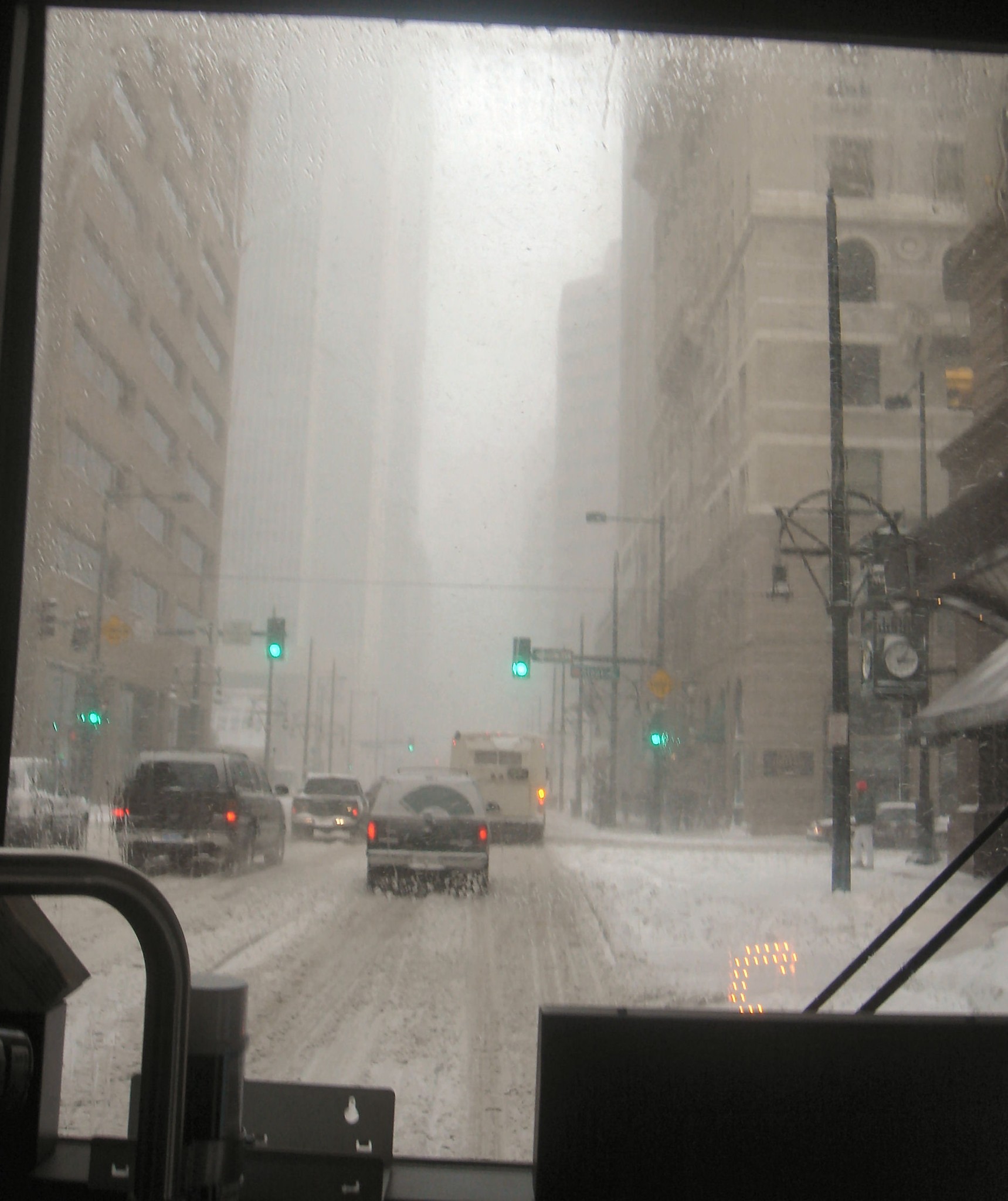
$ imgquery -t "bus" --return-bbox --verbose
[446,730,554,842]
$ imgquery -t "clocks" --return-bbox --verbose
[861,647,872,680]
[884,643,919,678]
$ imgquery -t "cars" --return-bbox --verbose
[2,757,92,850]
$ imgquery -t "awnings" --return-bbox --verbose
[916,641,1008,738]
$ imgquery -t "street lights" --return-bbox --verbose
[766,488,928,862]
[76,489,202,794]
[883,368,940,866]
[586,511,670,837]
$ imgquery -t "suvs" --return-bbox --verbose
[363,772,496,890]
[109,749,290,878]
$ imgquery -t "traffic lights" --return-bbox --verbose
[266,616,288,662]
[408,737,415,752]
[645,728,671,749]
[512,637,532,679]
[78,710,102,729]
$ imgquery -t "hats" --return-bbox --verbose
[856,780,867,791]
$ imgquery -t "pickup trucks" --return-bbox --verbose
[289,774,371,841]
